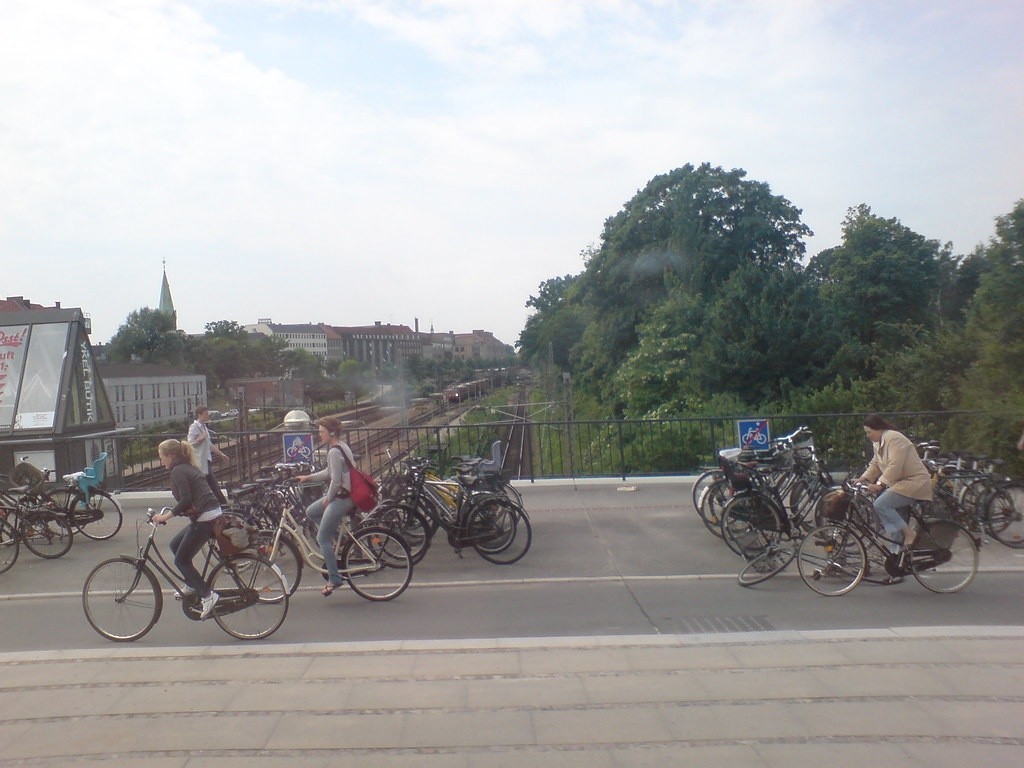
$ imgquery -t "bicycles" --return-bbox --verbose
[738,537,890,588]
[0,452,123,574]
[914,441,1024,547]
[82,505,291,642]
[234,478,414,604]
[201,441,534,569]
[797,474,981,597]
[691,422,868,556]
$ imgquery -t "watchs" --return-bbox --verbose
[877,481,885,487]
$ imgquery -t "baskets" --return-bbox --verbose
[8,462,44,494]
[819,486,853,522]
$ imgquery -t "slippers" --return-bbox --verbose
[321,585,335,596]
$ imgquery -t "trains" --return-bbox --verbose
[447,378,490,402]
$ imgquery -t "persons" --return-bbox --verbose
[849,415,934,554]
[294,416,358,593]
[186,405,230,504]
[150,439,224,620]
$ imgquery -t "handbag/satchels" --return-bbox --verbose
[328,446,380,513]
[213,515,250,553]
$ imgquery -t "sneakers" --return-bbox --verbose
[174,584,196,598]
[200,591,220,621]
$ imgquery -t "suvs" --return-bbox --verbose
[225,409,238,416]
[208,411,222,421]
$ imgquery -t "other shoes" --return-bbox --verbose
[884,577,904,583]
[900,543,913,552]
